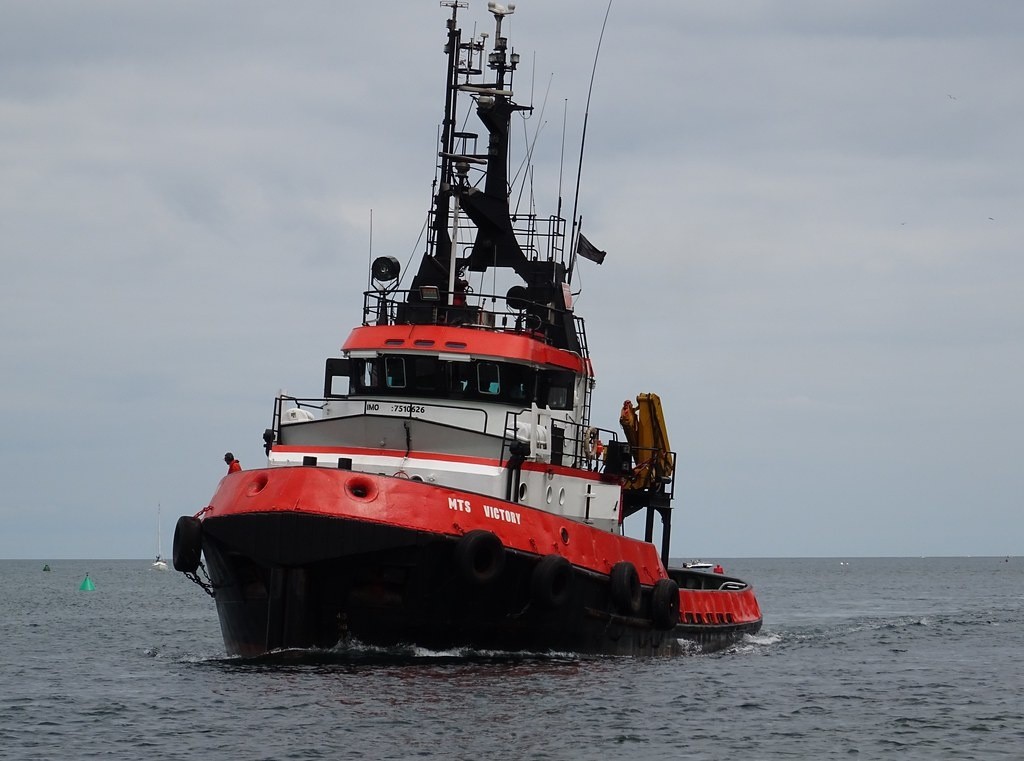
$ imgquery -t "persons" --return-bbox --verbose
[620,398,641,462]
[223,452,245,472]
[155,555,161,563]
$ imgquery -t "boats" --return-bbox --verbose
[167,0,761,660]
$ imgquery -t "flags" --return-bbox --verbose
[575,234,606,266]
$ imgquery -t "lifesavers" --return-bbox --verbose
[584,426,598,459]
